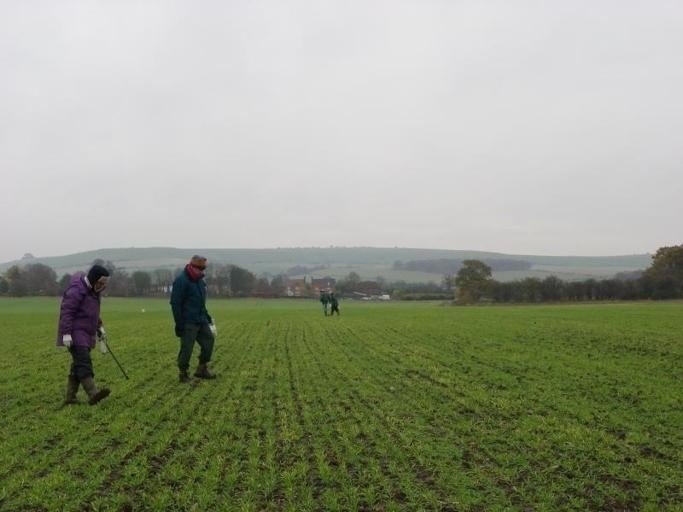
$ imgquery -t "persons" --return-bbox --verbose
[329,293,340,316]
[319,289,331,316]
[54,264,111,406]
[166,255,218,382]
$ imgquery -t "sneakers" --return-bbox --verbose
[195,362,212,378]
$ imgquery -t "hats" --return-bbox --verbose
[87,265,110,293]
[191,255,207,270]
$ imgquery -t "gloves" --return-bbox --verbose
[62,334,73,348]
[176,325,184,337]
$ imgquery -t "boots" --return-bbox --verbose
[82,374,110,405]
[67,374,81,403]
